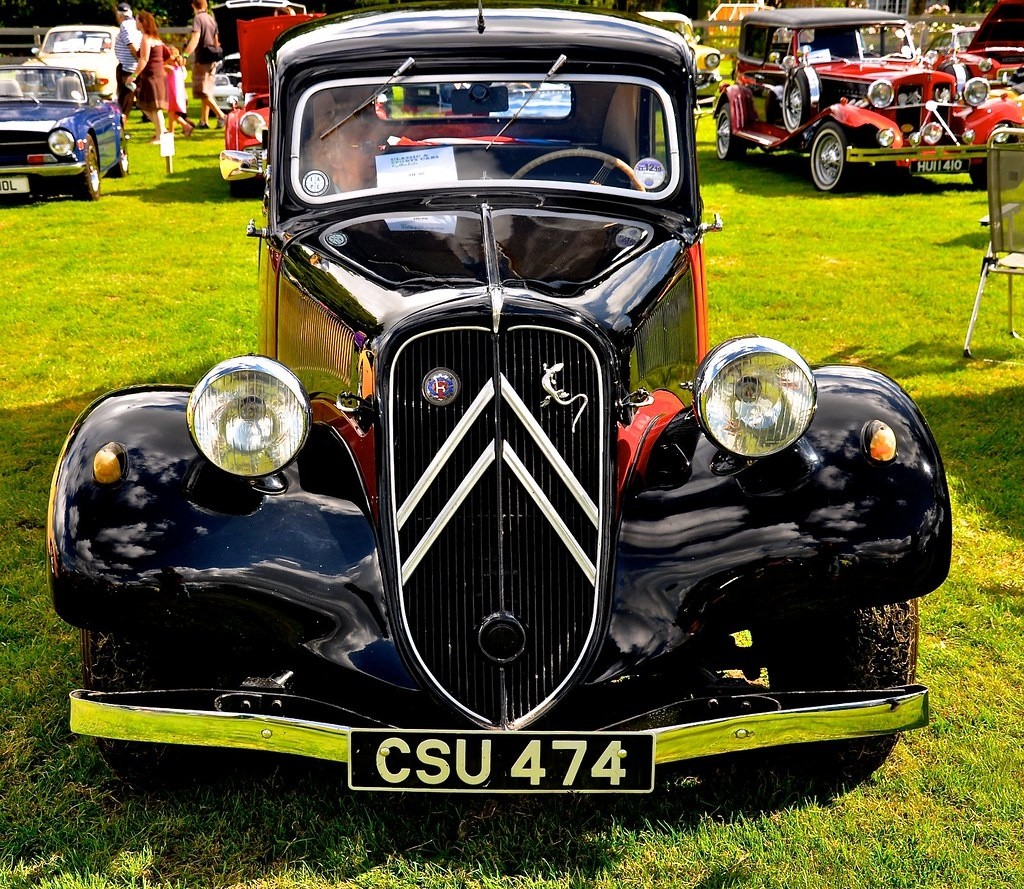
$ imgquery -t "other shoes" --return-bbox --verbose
[182,122,194,138]
[216,115,229,130]
[197,121,210,130]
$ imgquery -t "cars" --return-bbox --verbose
[206,52,245,119]
[219,14,391,197]
[13,24,121,105]
[628,7,724,108]
[927,0,1023,193]
[0,63,130,202]
[713,3,992,193]
[45,6,955,801]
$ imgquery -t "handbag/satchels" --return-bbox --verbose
[198,44,224,65]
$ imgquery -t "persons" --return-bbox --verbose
[308,105,381,195]
[114,0,228,144]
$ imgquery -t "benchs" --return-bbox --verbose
[386,144,629,184]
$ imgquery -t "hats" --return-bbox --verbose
[116,2,131,12]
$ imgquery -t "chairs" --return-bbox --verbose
[0,79,23,96]
[57,77,85,99]
[960,126,1024,362]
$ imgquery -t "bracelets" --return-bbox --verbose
[182,52,189,58]
[131,73,137,78]
